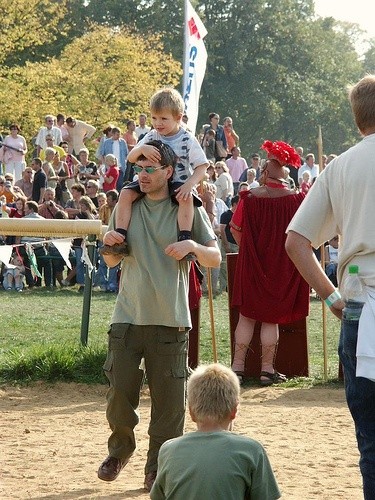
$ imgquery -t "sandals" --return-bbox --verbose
[235,371,244,385]
[260,369,288,386]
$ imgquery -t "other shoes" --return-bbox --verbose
[7,287,12,290]
[15,287,22,293]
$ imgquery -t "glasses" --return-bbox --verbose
[27,172,32,174]
[0,182,4,184]
[132,165,165,173]
[7,179,12,181]
[215,167,221,169]
[87,186,94,188]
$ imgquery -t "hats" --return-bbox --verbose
[262,140,301,184]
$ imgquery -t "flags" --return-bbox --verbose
[182,0,208,135]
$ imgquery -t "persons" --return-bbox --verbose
[285,76,374,500]
[151,363,281,500]
[99,141,221,492]
[0,111,152,291]
[183,110,262,299]
[301,171,311,196]
[228,140,309,385]
[102,88,210,258]
[324,235,339,280]
[284,146,336,190]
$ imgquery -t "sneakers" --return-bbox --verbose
[98,454,130,481]
[180,251,194,261]
[144,471,156,491]
[101,241,128,256]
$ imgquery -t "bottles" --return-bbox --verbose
[342,265,365,320]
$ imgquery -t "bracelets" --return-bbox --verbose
[324,290,342,308]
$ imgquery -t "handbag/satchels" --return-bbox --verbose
[216,141,227,157]
